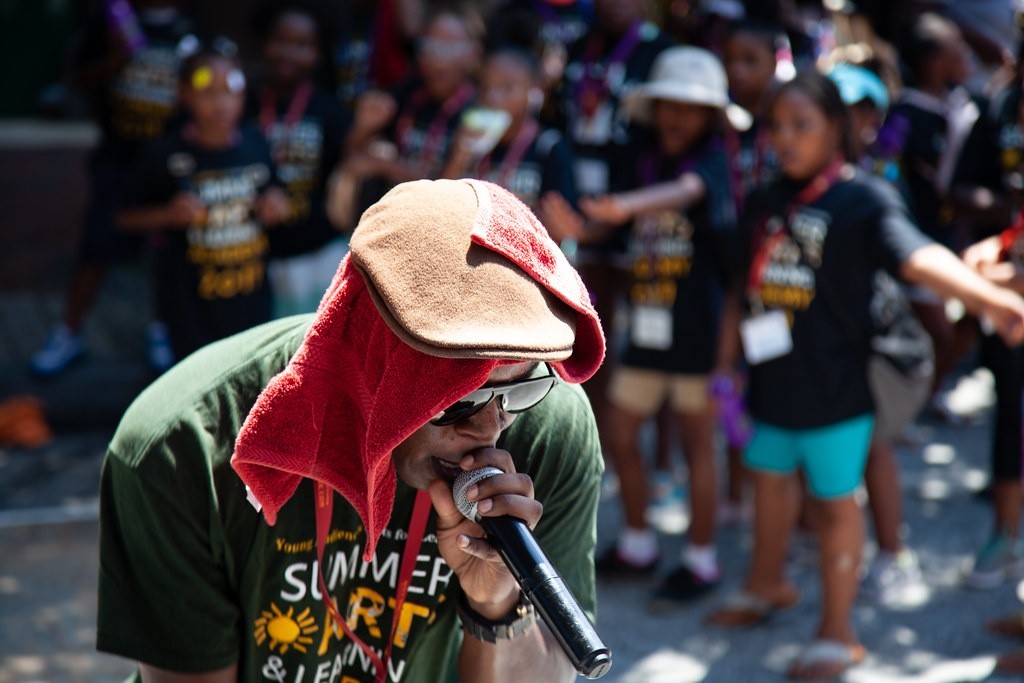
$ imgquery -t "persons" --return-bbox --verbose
[0,0,1024,683]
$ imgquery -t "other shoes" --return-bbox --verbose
[35,325,89,372]
[594,540,663,582]
[907,399,971,433]
[1,393,48,448]
[860,548,916,604]
[145,321,172,367]
[653,565,721,605]
[971,533,1024,589]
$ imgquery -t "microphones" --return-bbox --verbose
[452,466,613,680]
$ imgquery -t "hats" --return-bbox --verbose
[825,60,887,121]
[352,174,576,361]
[115,41,186,112]
[624,44,752,134]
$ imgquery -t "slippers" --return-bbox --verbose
[709,581,793,626]
[783,636,866,683]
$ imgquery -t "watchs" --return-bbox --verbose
[453,589,538,644]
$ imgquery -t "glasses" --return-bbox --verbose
[186,66,248,97]
[428,358,558,426]
